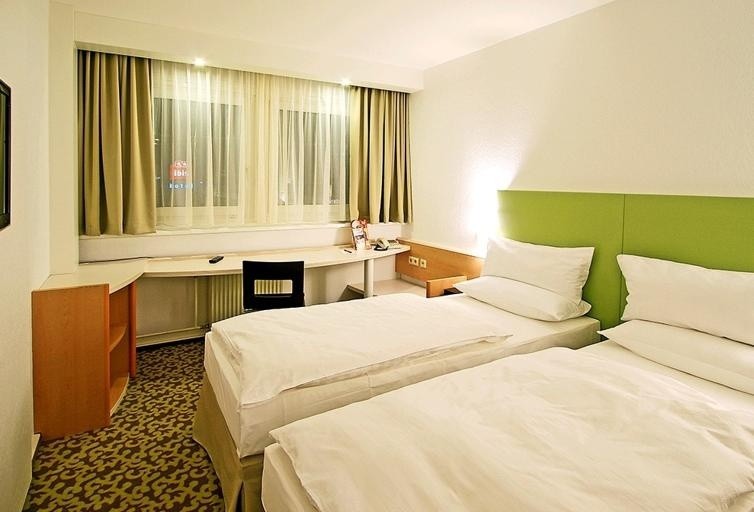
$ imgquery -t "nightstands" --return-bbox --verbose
[347,277,426,299]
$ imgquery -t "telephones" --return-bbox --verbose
[376,238,401,249]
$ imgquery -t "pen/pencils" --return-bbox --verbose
[344,249,352,253]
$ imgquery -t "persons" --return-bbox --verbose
[351,220,369,249]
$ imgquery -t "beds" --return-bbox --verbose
[192,293,601,512]
[259,339,754,512]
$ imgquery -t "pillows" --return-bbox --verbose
[616,254,754,346]
[481,233,595,306]
[596,320,754,395]
[452,275,592,321]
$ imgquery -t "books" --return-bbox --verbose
[350,218,372,251]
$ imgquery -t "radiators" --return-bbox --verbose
[207,274,283,328]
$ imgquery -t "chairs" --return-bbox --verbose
[243,261,304,311]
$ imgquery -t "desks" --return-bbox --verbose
[31,242,410,442]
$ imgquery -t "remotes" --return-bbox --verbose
[209,255,224,264]
